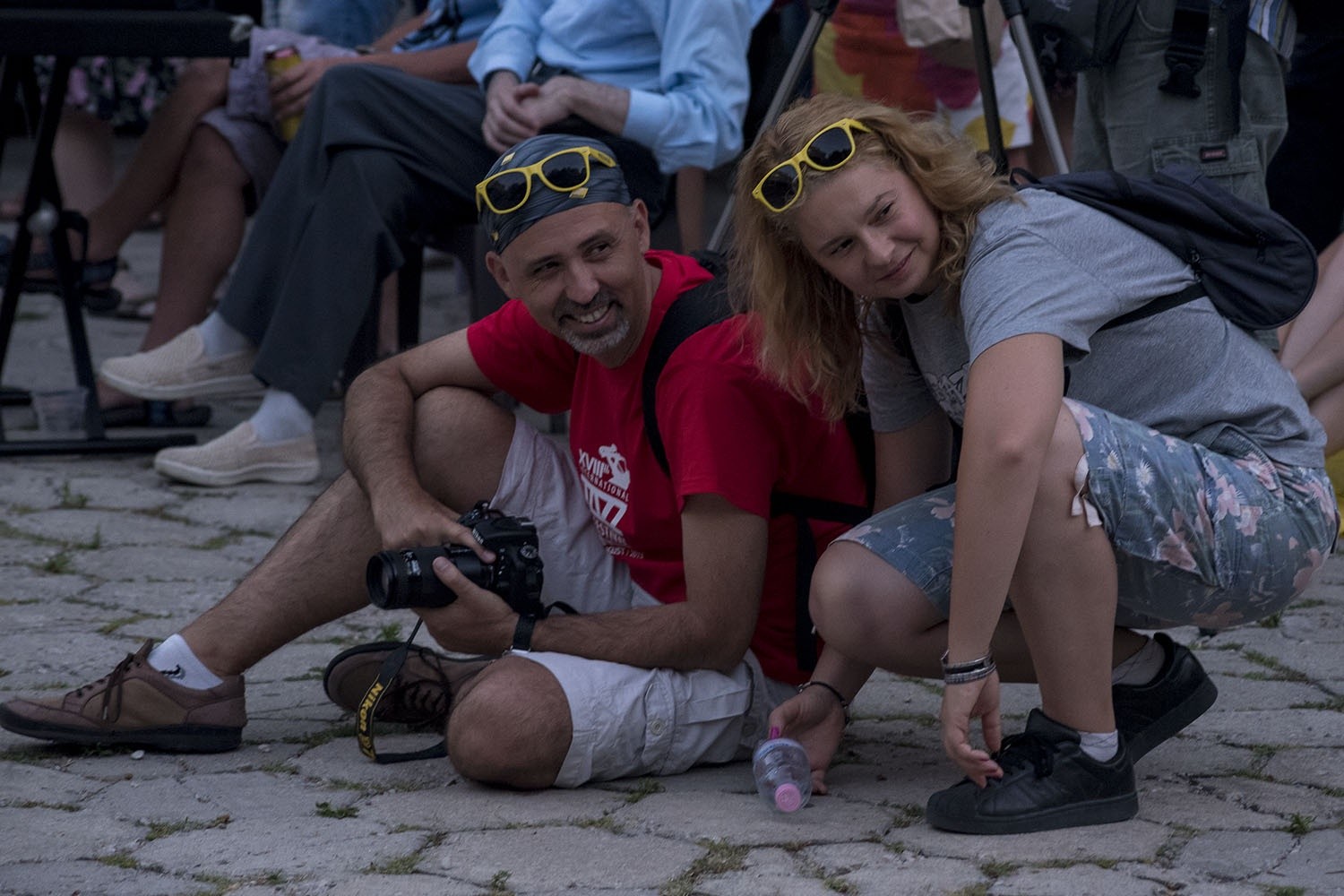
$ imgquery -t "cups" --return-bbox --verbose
[32,387,88,439]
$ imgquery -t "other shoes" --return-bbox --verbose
[18,245,117,291]
[152,418,322,486]
[99,325,267,401]
[89,399,213,427]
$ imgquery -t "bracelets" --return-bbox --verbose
[939,648,994,684]
[797,680,851,730]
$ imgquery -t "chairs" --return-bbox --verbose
[337,3,779,396]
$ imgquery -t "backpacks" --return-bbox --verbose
[979,157,1320,331]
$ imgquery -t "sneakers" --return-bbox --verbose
[0,638,248,753]
[1112,631,1219,760]
[924,708,1142,836]
[324,641,496,726]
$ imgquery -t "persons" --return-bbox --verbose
[726,91,1341,835]
[0,0,1344,486]
[0,134,871,790]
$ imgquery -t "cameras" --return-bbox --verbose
[365,500,543,620]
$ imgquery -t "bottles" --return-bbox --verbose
[752,733,813,813]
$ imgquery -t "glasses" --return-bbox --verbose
[476,144,617,213]
[751,118,874,212]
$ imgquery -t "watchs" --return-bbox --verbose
[501,613,537,656]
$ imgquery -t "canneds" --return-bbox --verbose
[264,43,306,143]
